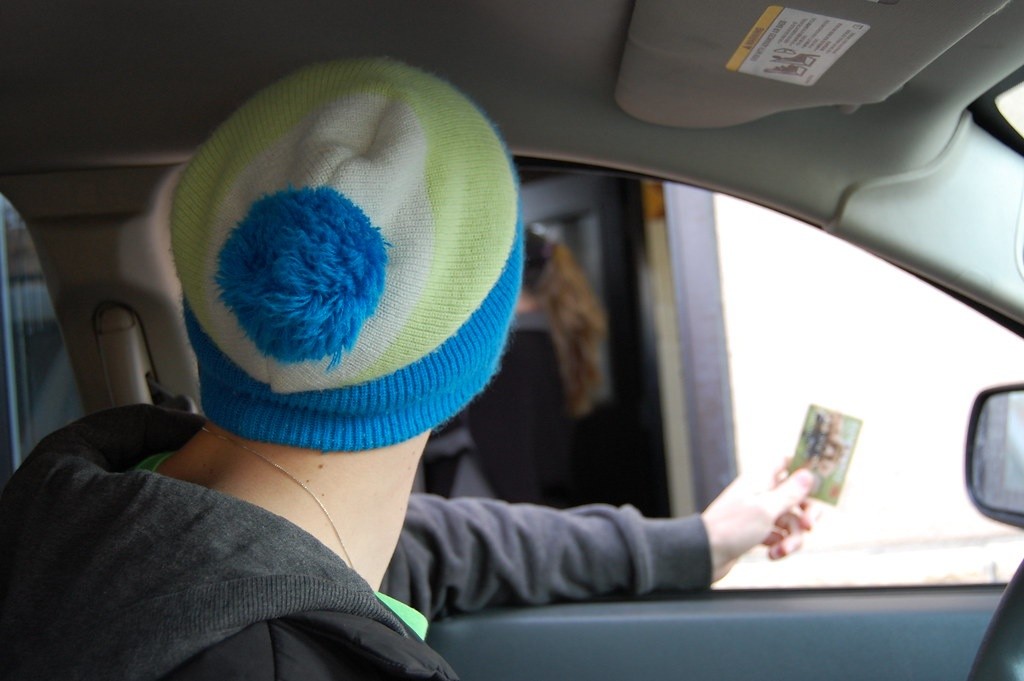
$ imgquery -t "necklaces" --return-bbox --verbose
[202,426,355,569]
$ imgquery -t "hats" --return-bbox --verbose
[170,57,524,451]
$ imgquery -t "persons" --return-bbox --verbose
[0,52,816,681]
